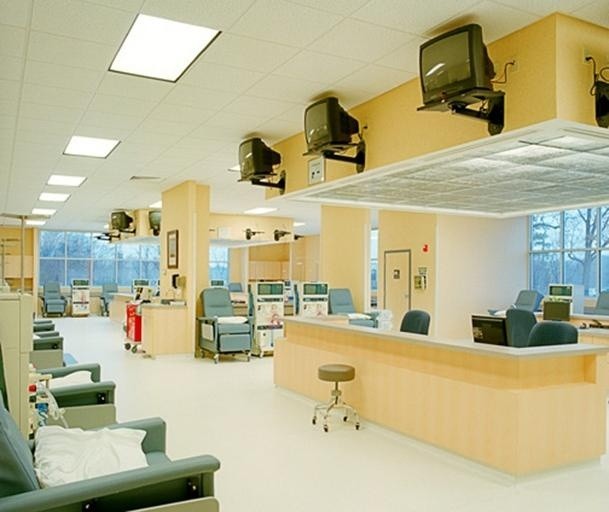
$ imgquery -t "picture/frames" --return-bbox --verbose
[307,157,327,185]
[166,229,180,270]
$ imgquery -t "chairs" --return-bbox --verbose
[198,285,252,363]
[41,280,68,316]
[398,309,430,338]
[30,331,63,368]
[0,390,221,512]
[330,286,375,329]
[28,364,117,427]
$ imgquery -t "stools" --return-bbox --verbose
[311,363,361,434]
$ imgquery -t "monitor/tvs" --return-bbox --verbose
[148,210,162,230]
[110,211,134,230]
[593,78,609,127]
[417,23,496,105]
[303,97,360,151]
[472,315,510,348]
[238,136,282,178]
